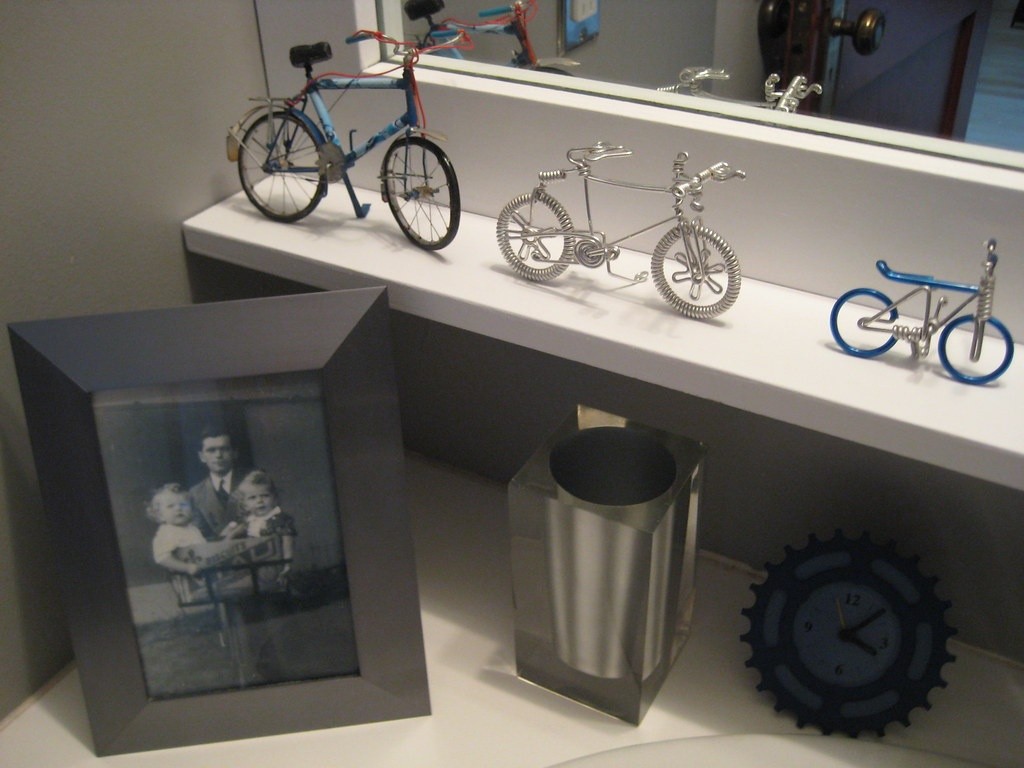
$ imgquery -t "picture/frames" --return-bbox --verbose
[8,286,430,757]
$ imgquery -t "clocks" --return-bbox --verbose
[740,527,958,740]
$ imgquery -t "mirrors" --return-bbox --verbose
[253,0,1024,344]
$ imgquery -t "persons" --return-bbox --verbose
[146,423,298,601]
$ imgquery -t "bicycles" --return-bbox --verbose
[830,239,1014,385]
[162,554,313,689]
[497,141,746,319]
[657,66,823,113]
[226,30,475,250]
[405,1,582,77]
